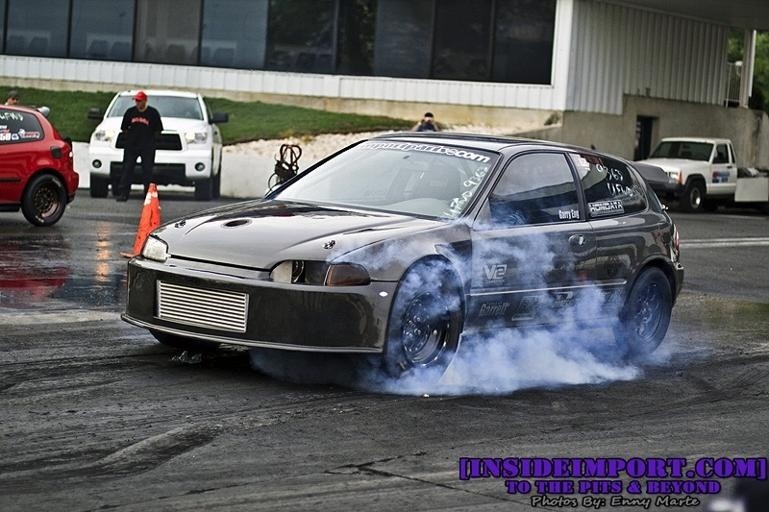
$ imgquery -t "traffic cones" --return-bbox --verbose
[120,183,160,258]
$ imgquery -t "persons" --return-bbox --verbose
[5,90,21,106]
[114,92,163,201]
[411,112,441,132]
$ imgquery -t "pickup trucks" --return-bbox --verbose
[637,137,769,213]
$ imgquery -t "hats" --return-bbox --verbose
[132,91,147,101]
[6,91,18,101]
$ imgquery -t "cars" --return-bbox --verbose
[88,90,228,200]
[0,105,79,227]
[121,132,684,388]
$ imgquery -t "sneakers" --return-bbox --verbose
[116,192,128,202]
